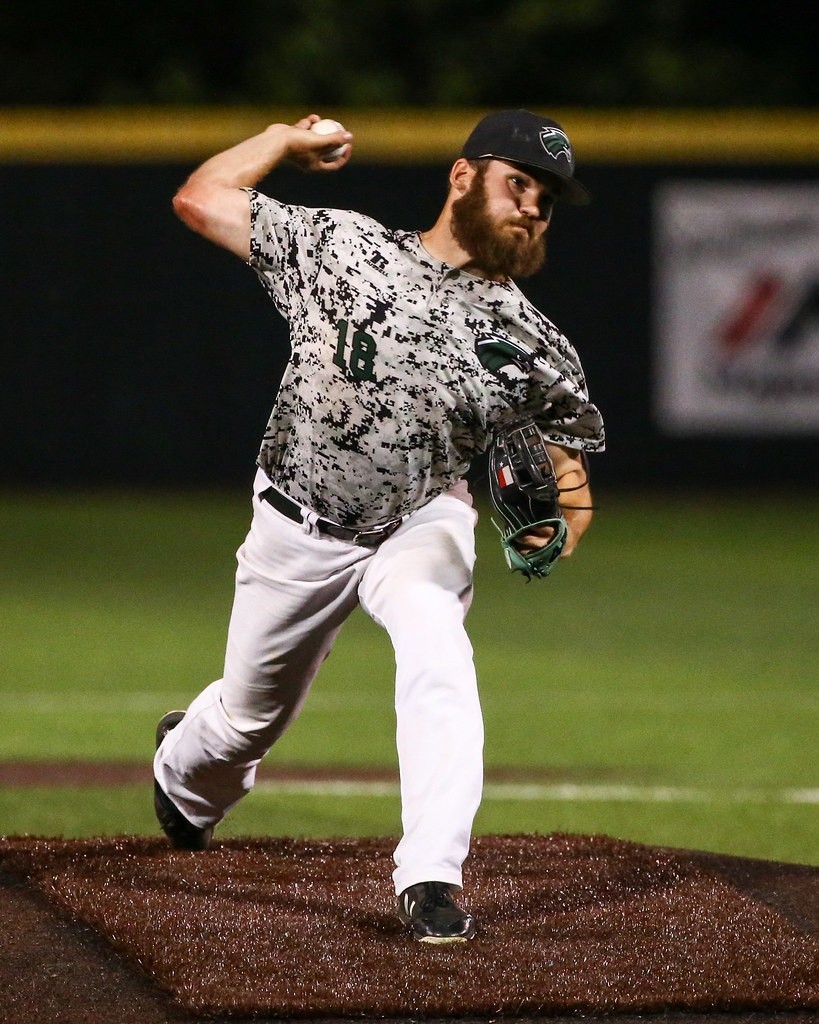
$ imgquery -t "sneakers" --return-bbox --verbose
[149,709,215,852]
[395,880,476,946]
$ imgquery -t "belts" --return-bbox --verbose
[259,486,403,548]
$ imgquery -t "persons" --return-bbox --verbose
[153,110,606,944]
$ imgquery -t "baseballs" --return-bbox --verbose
[308,120,351,161]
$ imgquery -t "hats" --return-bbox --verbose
[460,108,595,211]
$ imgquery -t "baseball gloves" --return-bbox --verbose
[486,416,601,586]
